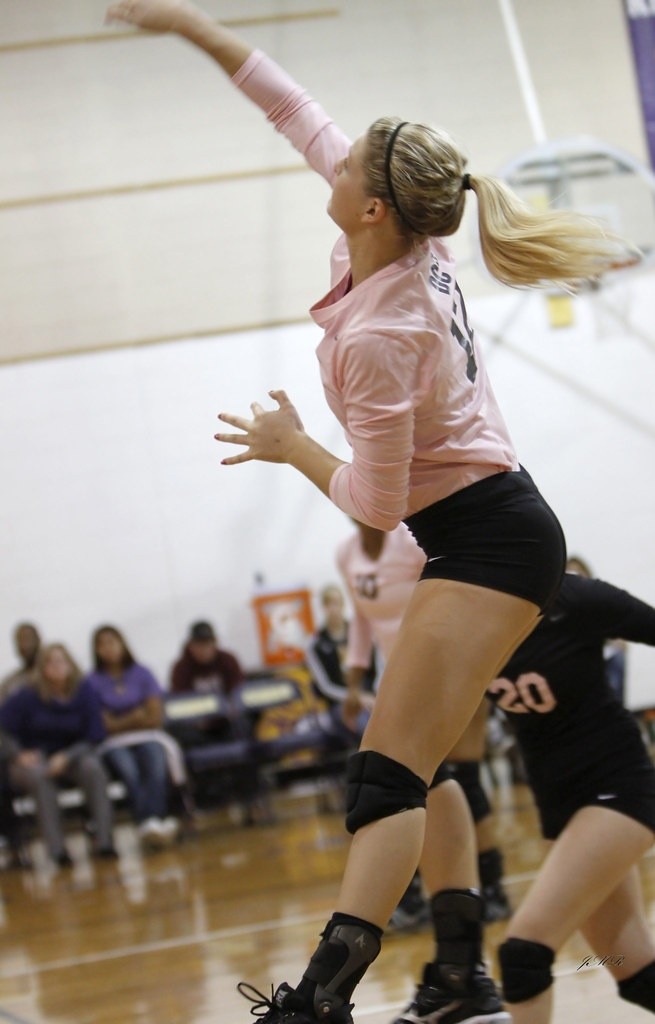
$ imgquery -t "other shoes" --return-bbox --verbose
[138,815,180,844]
[56,846,120,865]
[481,894,511,922]
[385,906,433,937]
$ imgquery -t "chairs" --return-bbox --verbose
[163,691,269,833]
[237,677,344,818]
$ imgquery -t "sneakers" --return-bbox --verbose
[256,981,355,1024]
[391,964,513,1023]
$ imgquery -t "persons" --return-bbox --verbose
[85,0,568,1023]
[564,557,627,712]
[0,621,65,866]
[481,573,654,1024]
[0,644,122,866]
